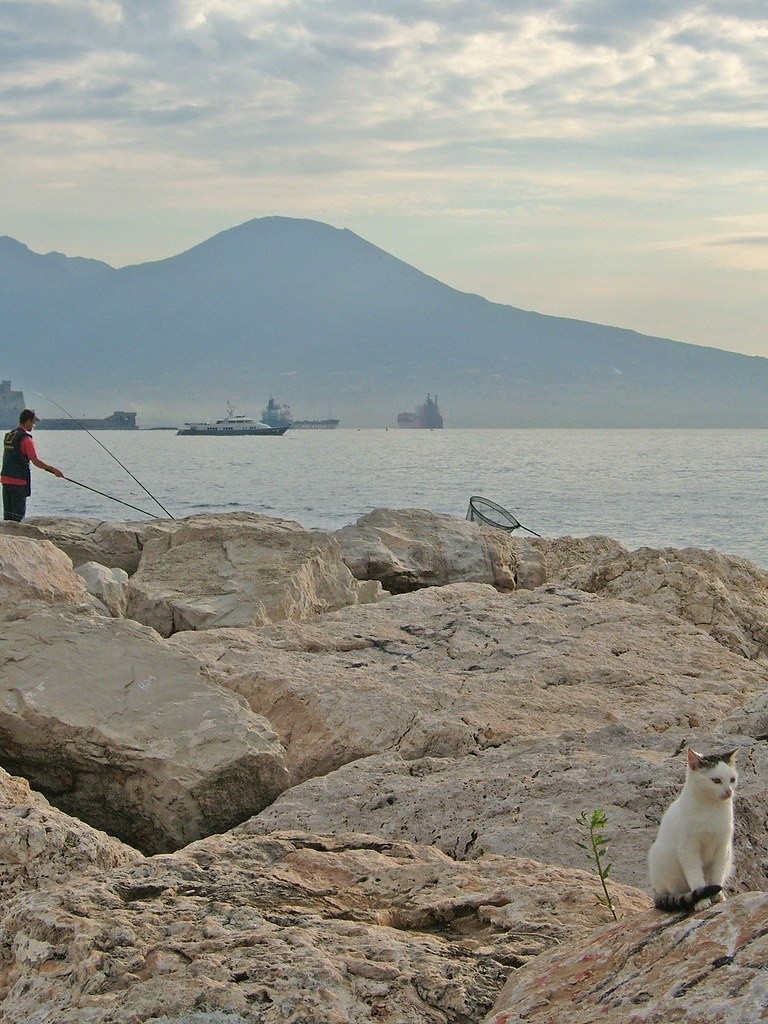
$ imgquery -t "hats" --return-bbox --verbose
[19,409,40,421]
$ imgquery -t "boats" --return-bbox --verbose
[259,398,340,430]
[35,411,139,431]
[397,393,443,430]
[176,409,290,436]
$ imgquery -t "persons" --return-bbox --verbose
[0,409,64,522]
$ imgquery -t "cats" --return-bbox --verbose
[647,746,740,913]
